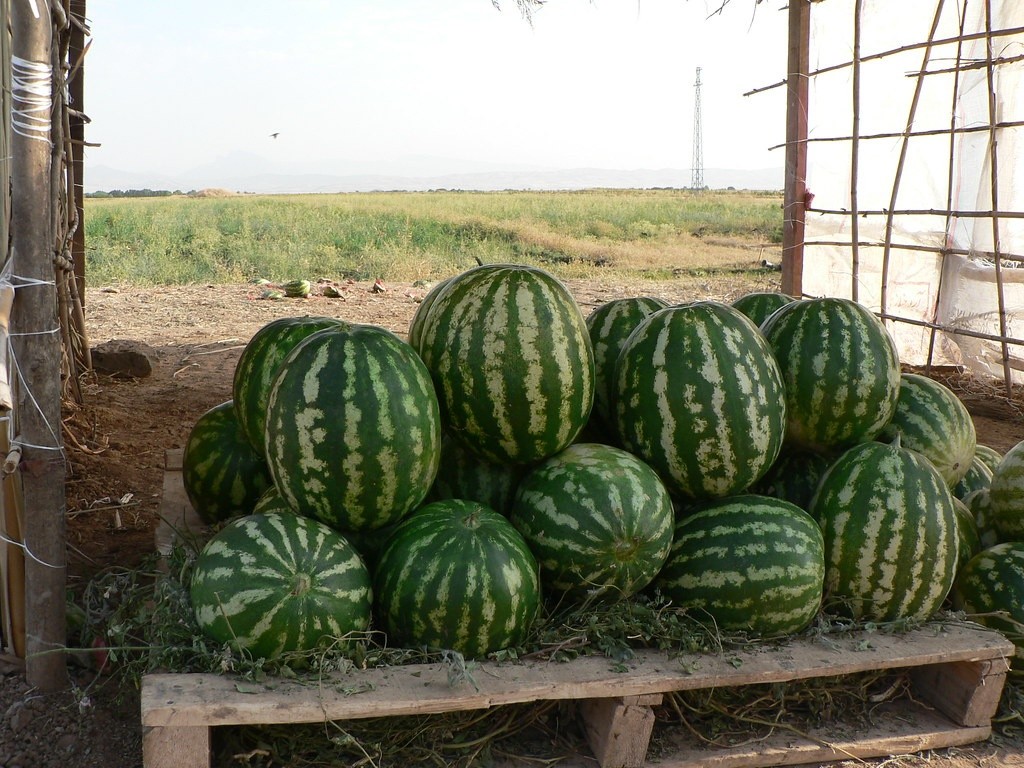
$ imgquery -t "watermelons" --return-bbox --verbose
[185,255,1024,680]
